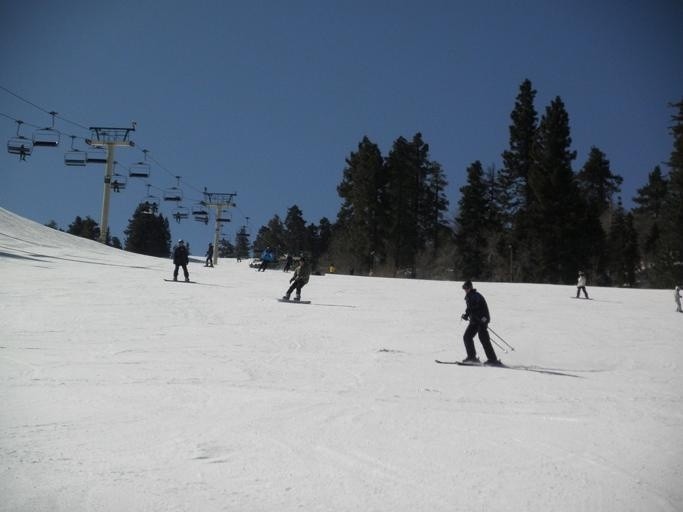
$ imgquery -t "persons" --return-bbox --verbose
[152,202,158,213]
[368,247,377,276]
[173,239,189,283]
[283,257,310,301]
[114,179,120,193]
[258,245,272,272]
[145,200,150,210]
[176,212,181,224]
[328,261,335,272]
[19,144,26,163]
[461,279,499,364]
[673,285,683,315]
[283,255,293,272]
[236,254,242,262]
[204,243,214,268]
[574,268,589,300]
[202,210,208,224]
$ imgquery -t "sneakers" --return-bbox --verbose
[282,296,300,302]
[173,278,190,282]
[462,356,500,365]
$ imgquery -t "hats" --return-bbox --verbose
[462,280,472,288]
[178,239,184,242]
[299,257,305,261]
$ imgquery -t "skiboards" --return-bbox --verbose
[435,361,536,369]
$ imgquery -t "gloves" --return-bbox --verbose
[462,314,487,322]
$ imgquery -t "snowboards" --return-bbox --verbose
[163,279,196,284]
[570,296,594,300]
[276,299,310,305]
